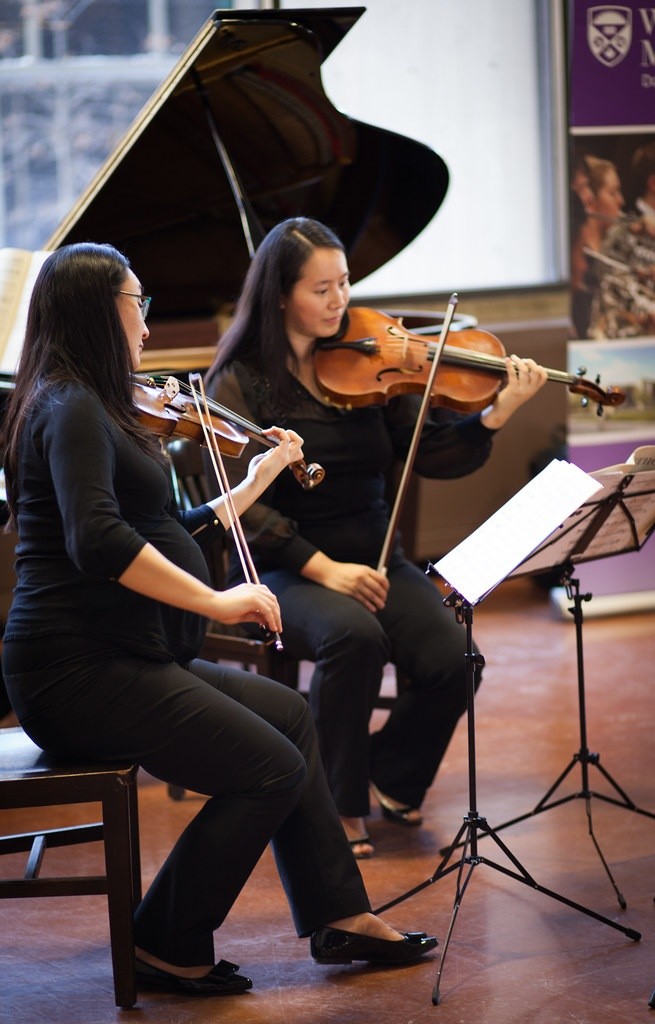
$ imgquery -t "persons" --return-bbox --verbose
[4,244,437,996]
[567,138,654,340]
[195,219,548,857]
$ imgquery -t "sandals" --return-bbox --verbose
[381,801,421,826]
[349,836,372,859]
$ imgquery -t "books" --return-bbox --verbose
[509,444,654,576]
[433,458,601,607]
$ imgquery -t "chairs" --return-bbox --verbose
[167,437,417,801]
[0,500,140,1008]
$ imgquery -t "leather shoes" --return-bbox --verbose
[310,925,437,964]
[135,957,252,996]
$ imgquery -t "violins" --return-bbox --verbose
[126,378,326,491]
[313,305,628,418]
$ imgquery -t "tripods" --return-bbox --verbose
[369,474,655,1006]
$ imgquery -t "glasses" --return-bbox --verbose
[113,290,151,320]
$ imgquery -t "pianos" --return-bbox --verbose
[1,3,478,567]
